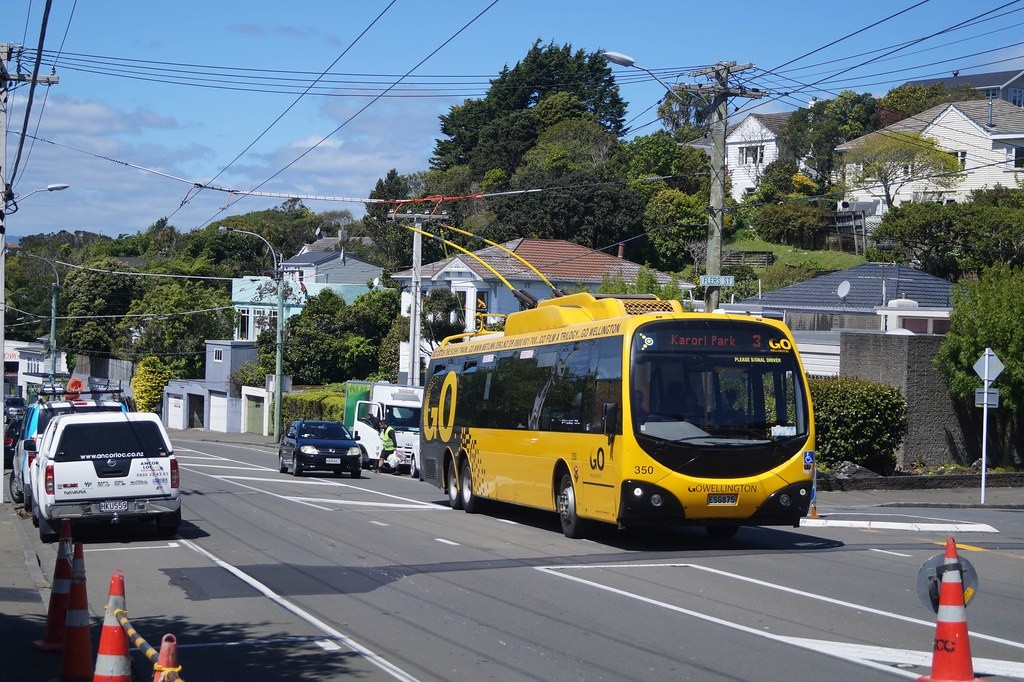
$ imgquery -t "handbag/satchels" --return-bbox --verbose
[387,450,406,468]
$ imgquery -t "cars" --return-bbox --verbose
[4,392,29,468]
[409,431,422,479]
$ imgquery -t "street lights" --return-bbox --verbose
[15,250,60,384]
[218,225,284,444]
[600,50,727,313]
[0,181,71,505]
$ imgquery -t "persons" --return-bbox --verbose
[304,427,315,437]
[372,419,401,476]
[635,390,648,418]
[681,389,704,415]
[366,412,380,430]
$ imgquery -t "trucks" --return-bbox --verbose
[342,379,425,469]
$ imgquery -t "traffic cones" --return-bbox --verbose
[33,517,73,656]
[91,567,133,682]
[914,536,990,682]
[55,542,95,682]
[152,633,179,682]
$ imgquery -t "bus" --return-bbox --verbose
[375,210,817,542]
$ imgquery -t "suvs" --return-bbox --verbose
[22,411,182,543]
[277,417,363,479]
[7,379,132,512]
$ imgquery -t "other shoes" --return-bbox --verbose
[392,470,401,475]
[371,468,382,474]
